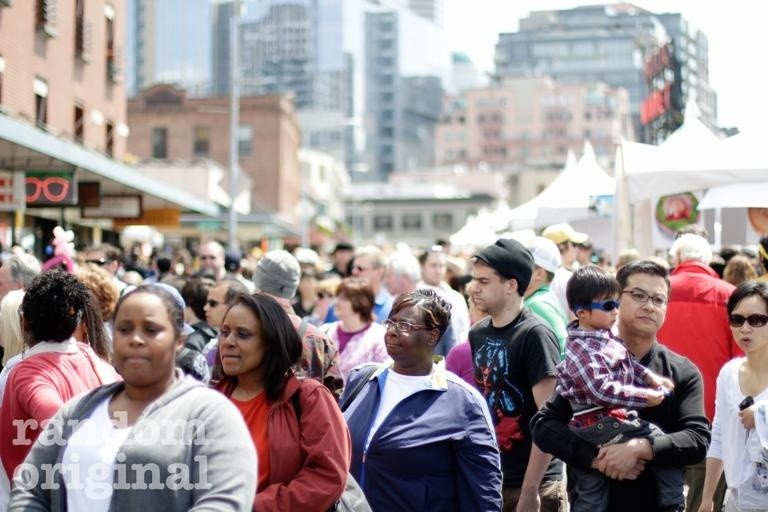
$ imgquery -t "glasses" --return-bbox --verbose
[317,292,323,299]
[728,314,768,328]
[85,259,112,266]
[205,299,225,306]
[201,255,216,261]
[620,290,668,305]
[382,319,432,332]
[17,304,23,320]
[573,300,620,316]
[351,264,366,271]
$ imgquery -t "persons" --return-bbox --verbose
[0,224,768,512]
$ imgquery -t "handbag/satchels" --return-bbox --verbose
[334,471,373,511]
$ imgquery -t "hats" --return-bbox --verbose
[540,223,589,244]
[252,250,301,299]
[224,250,242,272]
[470,238,534,297]
[519,236,562,274]
